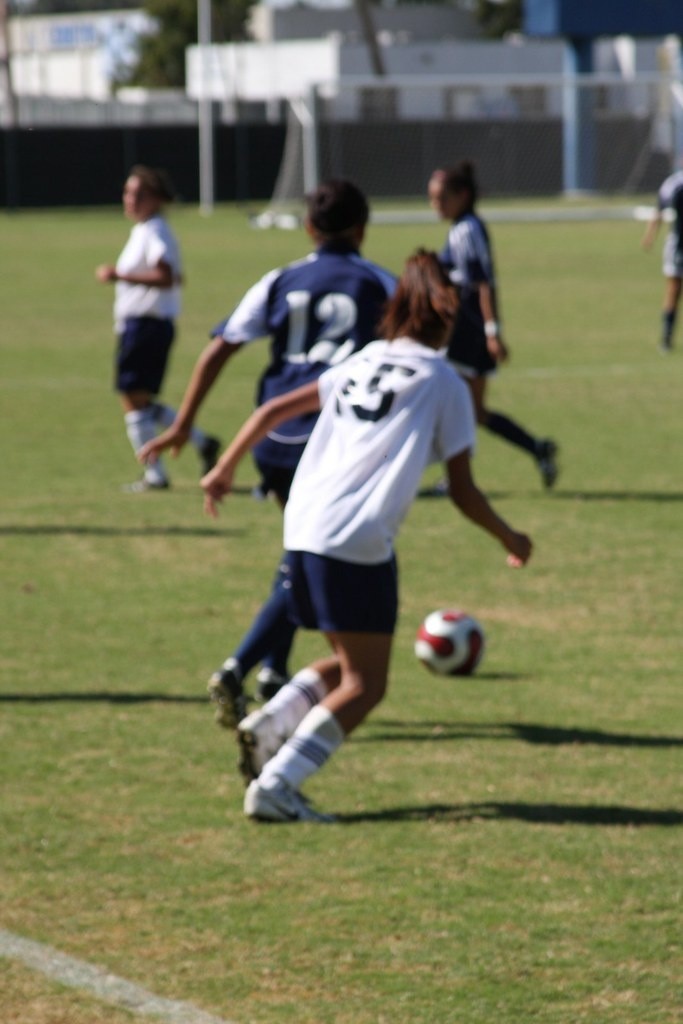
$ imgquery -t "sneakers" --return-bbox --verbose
[244,775,335,824]
[207,669,249,731]
[252,670,289,703]
[236,712,281,780]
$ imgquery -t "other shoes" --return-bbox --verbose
[538,438,557,488]
[659,331,671,351]
[200,437,220,475]
[126,479,168,494]
[430,478,448,496]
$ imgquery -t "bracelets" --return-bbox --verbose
[484,319,499,339]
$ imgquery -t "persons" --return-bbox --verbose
[93,160,219,492]
[641,168,682,350]
[198,242,530,821]
[134,174,403,728]
[417,160,558,498]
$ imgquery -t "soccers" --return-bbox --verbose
[414,607,487,674]
